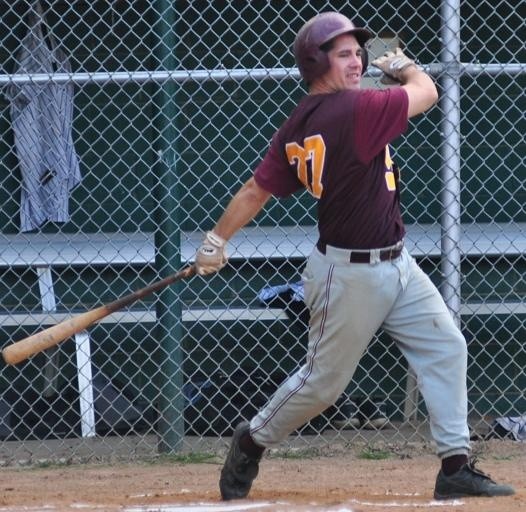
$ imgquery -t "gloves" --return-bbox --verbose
[194,231,227,276]
[372,48,415,85]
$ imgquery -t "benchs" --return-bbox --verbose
[0,222,526,440]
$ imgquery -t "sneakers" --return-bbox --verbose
[334,397,361,429]
[218,418,269,504]
[361,396,390,430]
[432,455,519,501]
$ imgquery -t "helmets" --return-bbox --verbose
[292,12,376,82]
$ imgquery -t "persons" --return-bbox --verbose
[197,13,516,499]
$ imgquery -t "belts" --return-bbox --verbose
[315,239,404,264]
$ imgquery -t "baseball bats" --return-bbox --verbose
[1,253,227,365]
